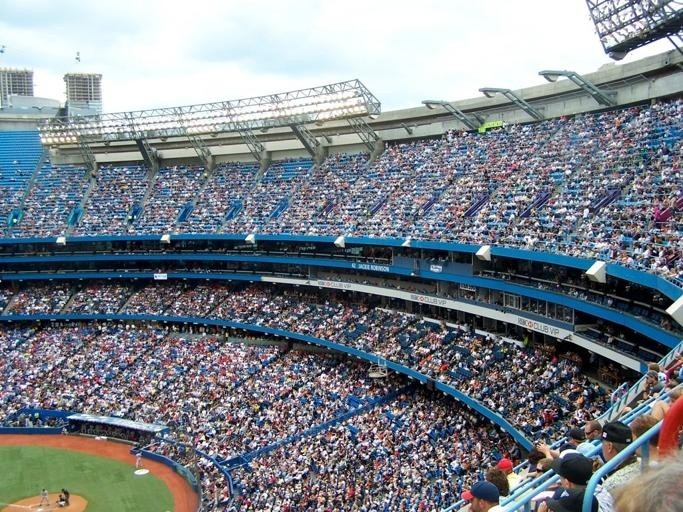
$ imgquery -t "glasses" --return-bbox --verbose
[536,469,545,473]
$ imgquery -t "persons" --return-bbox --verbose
[0,94,682,512]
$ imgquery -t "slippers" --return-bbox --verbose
[586,429,598,435]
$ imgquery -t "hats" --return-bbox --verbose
[461,482,499,503]
[658,396,683,459]
[496,459,512,471]
[565,429,585,440]
[560,444,576,452]
[550,453,592,484]
[601,422,632,443]
